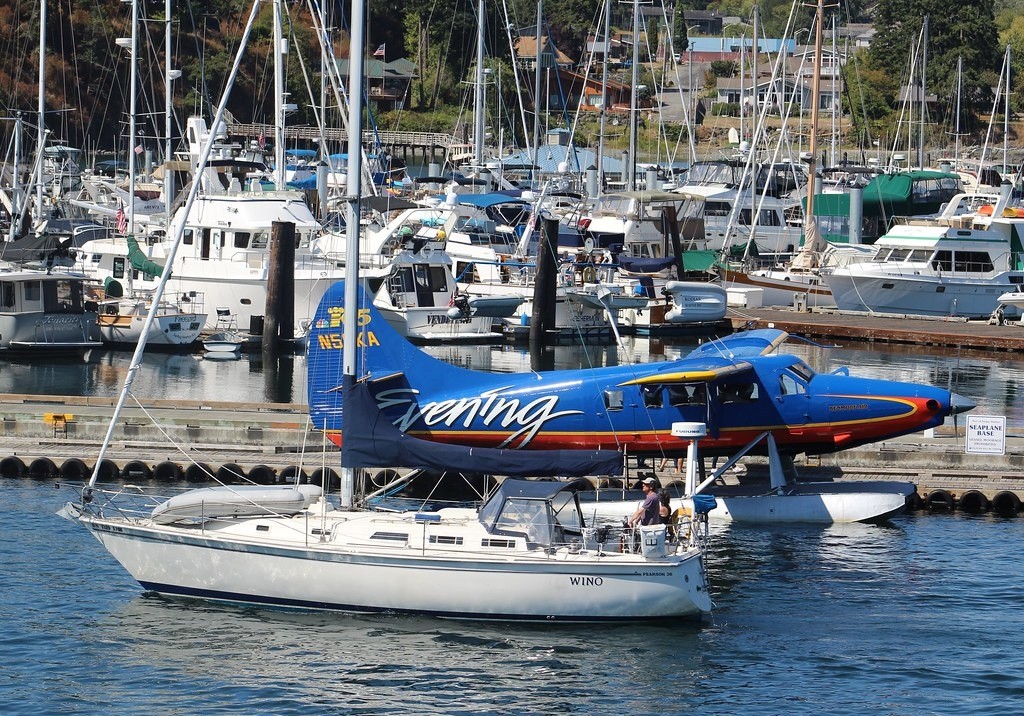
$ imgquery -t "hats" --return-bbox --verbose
[641,477,656,484]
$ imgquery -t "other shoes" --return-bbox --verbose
[733,466,743,473]
[656,467,663,472]
[711,468,718,473]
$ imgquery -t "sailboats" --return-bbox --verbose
[59,1,714,629]
[0,0,1024,368]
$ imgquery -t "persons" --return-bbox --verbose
[711,457,744,473]
[659,494,672,524]
[693,385,707,403]
[656,458,684,474]
[557,251,573,276]
[569,255,583,272]
[595,256,604,264]
[637,455,649,468]
[628,477,660,528]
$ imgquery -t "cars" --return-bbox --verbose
[670,53,682,66]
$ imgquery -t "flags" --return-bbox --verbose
[373,43,385,95]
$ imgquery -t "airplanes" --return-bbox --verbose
[306,277,979,528]
